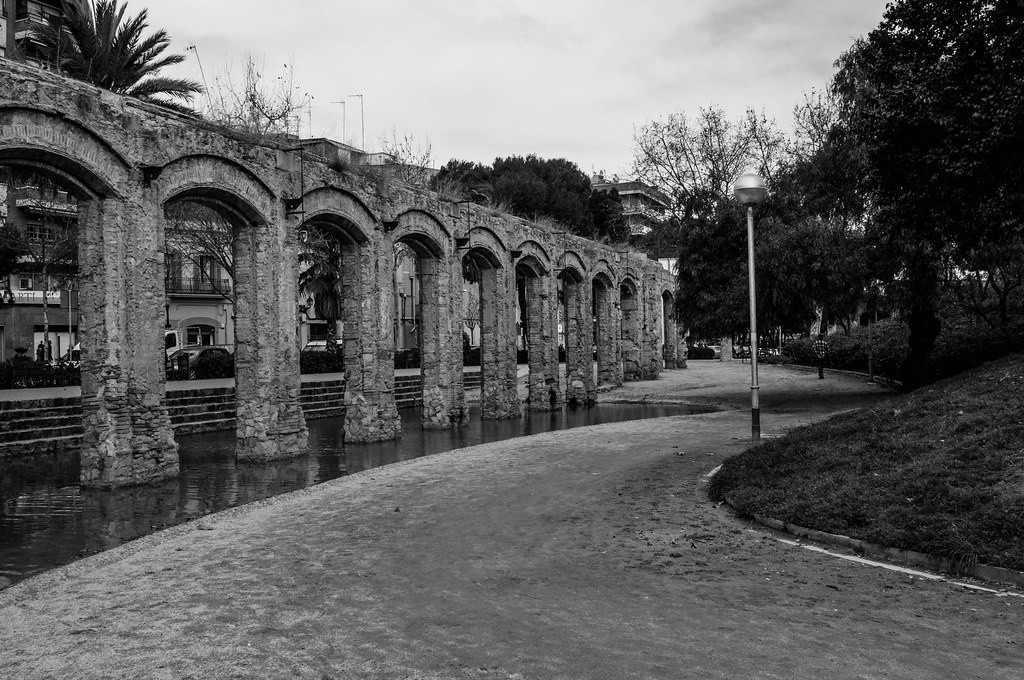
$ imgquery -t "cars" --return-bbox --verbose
[301,340,343,351]
[63,342,81,367]
[166,347,230,380]
[705,338,780,358]
[165,330,179,354]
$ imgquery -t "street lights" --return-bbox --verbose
[734,174,767,441]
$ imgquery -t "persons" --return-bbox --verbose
[812,334,829,380]
[36,340,53,362]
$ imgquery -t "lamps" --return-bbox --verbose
[380,221,399,232]
[299,295,314,313]
[142,166,166,188]
[281,197,307,215]
[509,250,523,260]
